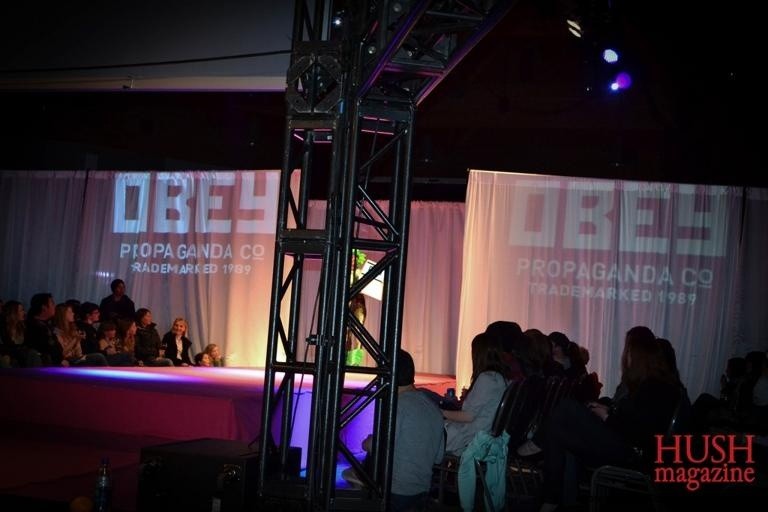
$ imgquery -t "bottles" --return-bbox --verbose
[92,455,114,512]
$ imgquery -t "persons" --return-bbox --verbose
[341,320,768,512]
[0,278,227,368]
[339,247,367,366]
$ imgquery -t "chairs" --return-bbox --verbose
[431,371,727,511]
